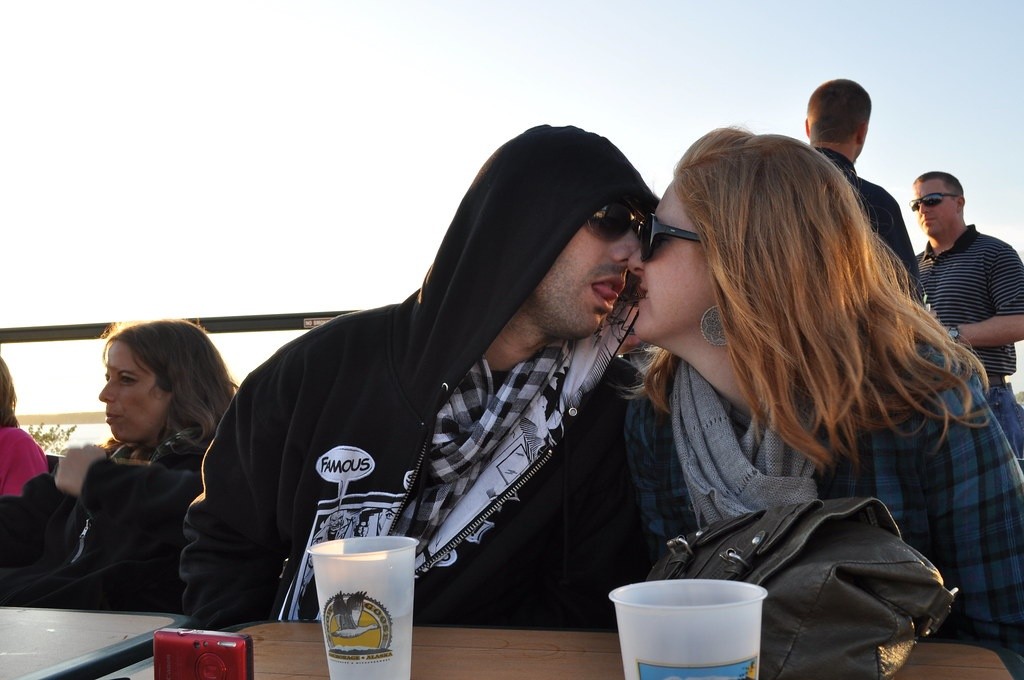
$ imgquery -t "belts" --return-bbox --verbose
[985,375,1006,387]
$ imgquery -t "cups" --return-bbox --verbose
[307,536,420,680]
[608,579,768,680]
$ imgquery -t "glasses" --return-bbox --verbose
[910,192,960,212]
[583,197,647,241]
[640,213,703,263]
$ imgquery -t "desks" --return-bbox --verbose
[97,619,1024,680]
[0,606,199,680]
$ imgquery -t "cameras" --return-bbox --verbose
[152,628,254,680]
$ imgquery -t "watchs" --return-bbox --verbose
[948,325,960,342]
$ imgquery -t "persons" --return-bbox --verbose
[908,171,1024,460]
[0,322,234,608]
[0,358,48,494]
[803,78,920,290]
[626,129,1022,654]
[179,127,654,630]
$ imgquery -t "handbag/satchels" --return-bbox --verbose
[646,493,958,680]
